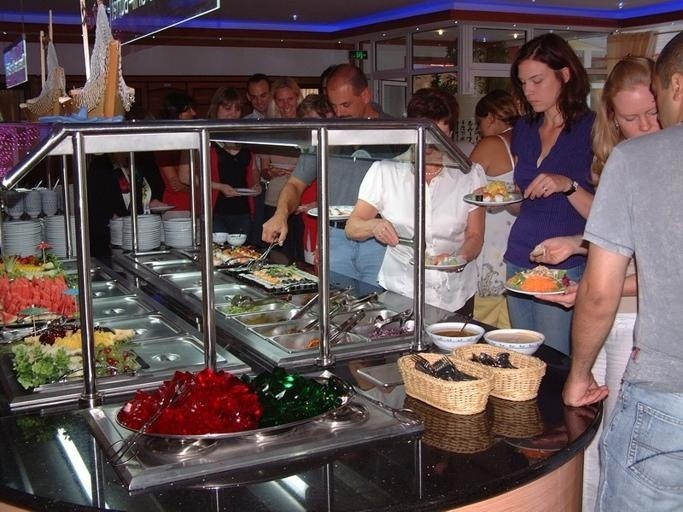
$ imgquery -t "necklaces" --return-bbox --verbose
[425,167,443,175]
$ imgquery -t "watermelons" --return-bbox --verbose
[0,272,79,326]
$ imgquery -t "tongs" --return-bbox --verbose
[397,236,415,248]
[289,284,414,346]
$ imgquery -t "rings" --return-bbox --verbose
[542,186,547,192]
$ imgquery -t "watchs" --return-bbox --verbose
[562,178,577,196]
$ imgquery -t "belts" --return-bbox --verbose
[328,219,347,229]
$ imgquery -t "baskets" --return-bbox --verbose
[484,394,547,440]
[396,352,497,416]
[453,342,549,403]
[401,394,494,457]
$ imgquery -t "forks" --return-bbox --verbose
[410,353,463,381]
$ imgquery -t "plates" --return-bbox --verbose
[463,189,526,209]
[504,278,579,295]
[307,205,357,220]
[109,204,205,251]
[408,255,469,271]
[234,187,262,196]
[0,215,75,260]
[269,162,297,172]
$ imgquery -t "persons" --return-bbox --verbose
[473,31,598,358]
[464,88,514,331]
[344,89,485,320]
[558,30,682,512]
[87,63,409,287]
[527,53,664,512]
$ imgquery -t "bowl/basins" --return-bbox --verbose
[424,317,486,351]
[483,325,546,357]
[226,233,248,247]
[5,189,58,219]
[209,231,229,246]
